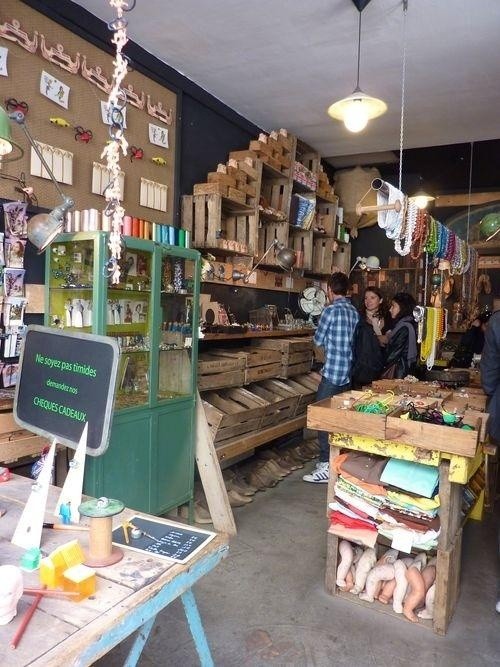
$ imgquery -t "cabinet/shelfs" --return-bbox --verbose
[41,227,200,526]
[184,258,351,487]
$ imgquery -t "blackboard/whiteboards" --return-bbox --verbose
[13,324,120,458]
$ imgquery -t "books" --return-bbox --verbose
[0,231,6,268]
[2,296,29,327]
[2,267,26,297]
[3,199,29,238]
[2,362,20,388]
[4,324,27,358]
[4,238,27,269]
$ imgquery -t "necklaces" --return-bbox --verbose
[374,179,482,308]
[412,305,450,373]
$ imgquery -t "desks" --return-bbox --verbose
[0,472,230,667]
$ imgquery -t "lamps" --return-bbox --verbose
[326,1,390,134]
[408,174,436,210]
[8,108,74,252]
[478,213,500,243]
[232,238,299,285]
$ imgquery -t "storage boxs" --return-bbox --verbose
[179,129,353,278]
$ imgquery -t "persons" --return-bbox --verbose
[378,290,421,382]
[298,269,362,485]
[361,284,396,373]
[479,291,499,514]
[124,303,132,323]
[63,297,122,327]
[450,308,494,367]
[132,303,144,322]
[7,240,25,268]
[349,292,390,389]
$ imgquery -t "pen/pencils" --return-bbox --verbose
[43,523,90,531]
[10,585,80,650]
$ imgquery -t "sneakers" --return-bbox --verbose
[302,461,329,484]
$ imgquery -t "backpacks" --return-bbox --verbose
[346,301,385,386]
